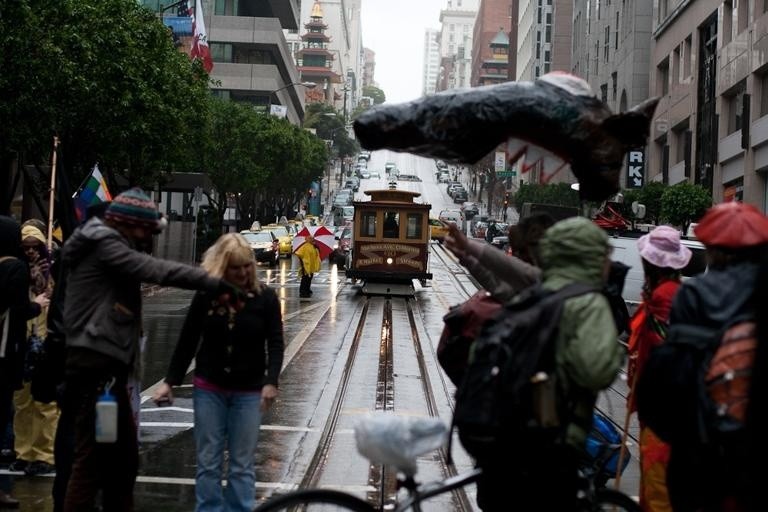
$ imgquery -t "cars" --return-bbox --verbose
[430,159,511,249]
[329,188,355,271]
[343,151,422,190]
[237,212,321,268]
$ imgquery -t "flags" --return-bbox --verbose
[189,1,214,74]
[74,162,113,221]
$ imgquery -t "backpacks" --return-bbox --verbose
[686,273,757,431]
[450,281,631,465]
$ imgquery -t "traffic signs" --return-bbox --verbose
[497,171,516,175]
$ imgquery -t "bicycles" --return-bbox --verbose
[252,405,641,512]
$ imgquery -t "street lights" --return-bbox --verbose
[339,84,351,188]
[265,80,317,113]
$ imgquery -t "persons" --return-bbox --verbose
[1,186,285,512]
[383,211,400,237]
[294,234,320,298]
[437,201,767,512]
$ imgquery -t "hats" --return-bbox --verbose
[695,200,768,248]
[636,224,694,270]
[104,187,168,230]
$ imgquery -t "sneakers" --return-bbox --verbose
[2,448,52,474]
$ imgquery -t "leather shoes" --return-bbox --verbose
[0,489,20,509]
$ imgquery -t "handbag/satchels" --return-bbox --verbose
[31,334,64,402]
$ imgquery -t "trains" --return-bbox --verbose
[346,188,434,298]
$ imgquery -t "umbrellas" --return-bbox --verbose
[293,225,337,262]
